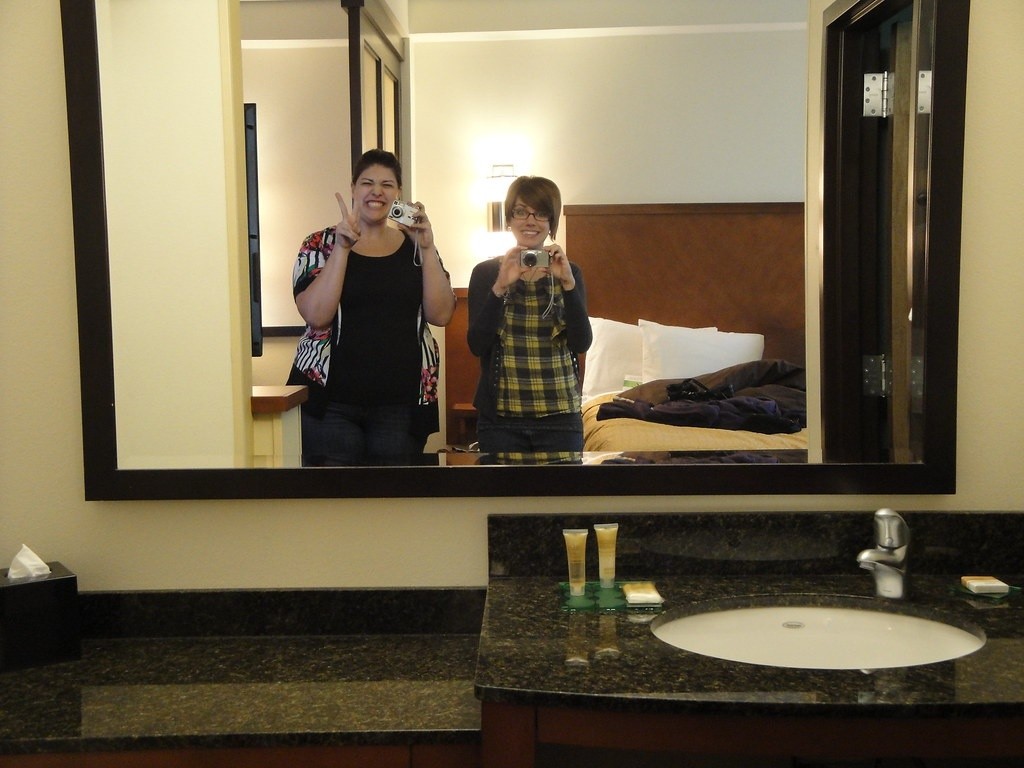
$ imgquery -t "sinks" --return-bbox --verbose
[649,591,988,671]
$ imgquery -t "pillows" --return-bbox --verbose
[581,316,718,407]
[638,319,764,385]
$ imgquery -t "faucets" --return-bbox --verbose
[856,507,913,599]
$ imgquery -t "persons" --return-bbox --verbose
[287,149,456,464]
[466,175,593,450]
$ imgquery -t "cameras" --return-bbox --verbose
[388,200,420,228]
[520,250,550,268]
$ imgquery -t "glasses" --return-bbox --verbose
[511,209,550,221]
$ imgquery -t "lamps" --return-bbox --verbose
[481,164,519,232]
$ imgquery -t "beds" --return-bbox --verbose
[576,389,808,452]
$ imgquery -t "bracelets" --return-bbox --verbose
[492,285,509,298]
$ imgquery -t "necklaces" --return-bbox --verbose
[522,268,538,280]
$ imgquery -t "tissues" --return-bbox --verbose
[0,547,85,672]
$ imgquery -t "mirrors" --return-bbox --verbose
[61,0,971,502]
